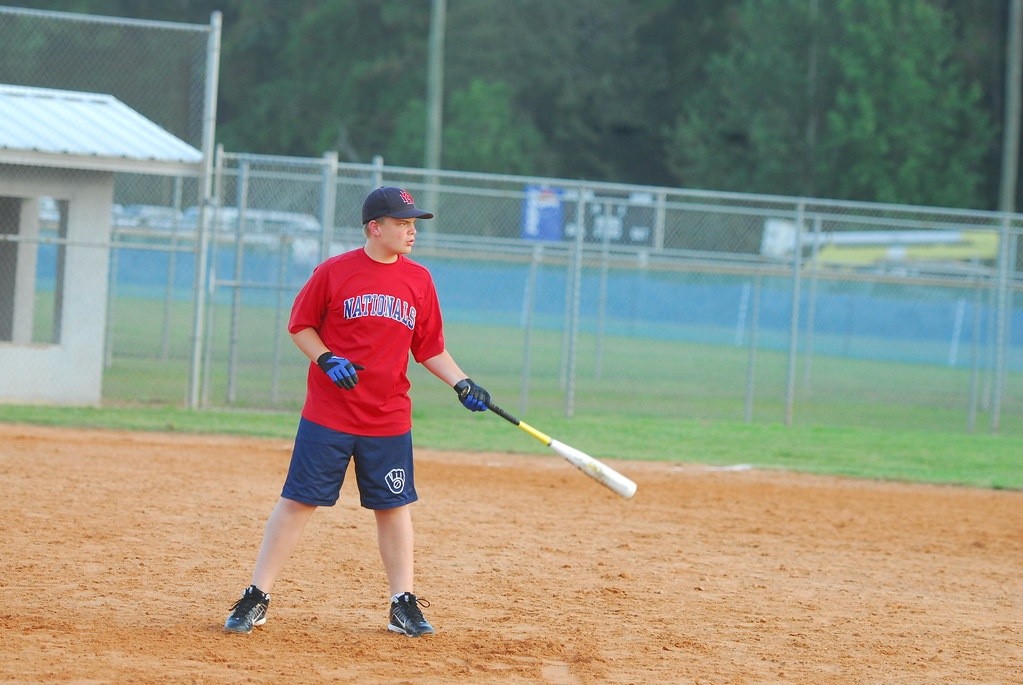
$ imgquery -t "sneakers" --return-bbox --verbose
[225,585,270,634]
[388,592,435,637]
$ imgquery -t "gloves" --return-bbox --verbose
[317,351,365,390]
[454,378,490,412]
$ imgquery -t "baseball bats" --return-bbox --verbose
[460,385,639,500]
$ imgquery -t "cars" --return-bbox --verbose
[39,197,318,235]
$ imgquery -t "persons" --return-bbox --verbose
[225,186,490,637]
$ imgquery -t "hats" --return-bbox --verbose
[362,186,433,225]
